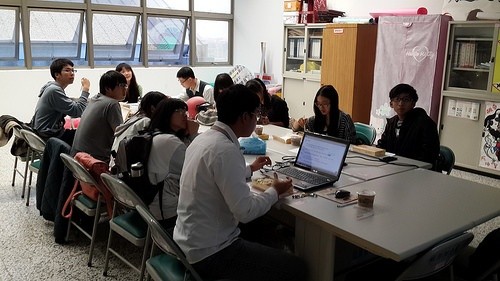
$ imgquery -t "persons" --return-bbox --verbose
[377,83,442,175]
[29,58,91,147]
[176,65,215,106]
[127,97,188,232]
[70,70,128,165]
[108,89,168,197]
[291,84,358,145]
[116,63,143,101]
[172,73,310,281]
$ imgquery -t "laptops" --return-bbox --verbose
[264,132,351,192]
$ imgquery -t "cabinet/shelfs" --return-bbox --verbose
[282,23,324,120]
[370,14,453,145]
[436,20,500,178]
[321,23,378,124]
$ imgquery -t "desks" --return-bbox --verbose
[199,123,500,281]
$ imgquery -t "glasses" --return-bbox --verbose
[314,102,331,108]
[176,109,187,117]
[178,77,189,83]
[392,96,412,102]
[63,69,77,73]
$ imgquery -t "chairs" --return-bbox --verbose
[351,121,377,146]
[445,228,500,281]
[10,126,43,199]
[343,233,475,281]
[19,128,47,206]
[99,171,175,281]
[59,152,119,266]
[135,203,203,281]
[439,145,456,174]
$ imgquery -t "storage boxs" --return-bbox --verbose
[281,0,326,24]
[352,144,385,156]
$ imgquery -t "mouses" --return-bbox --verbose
[335,189,350,197]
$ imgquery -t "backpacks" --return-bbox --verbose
[112,130,164,205]
[61,152,113,218]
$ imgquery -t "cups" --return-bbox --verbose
[357,190,376,208]
[255,126,263,135]
[291,136,301,146]
